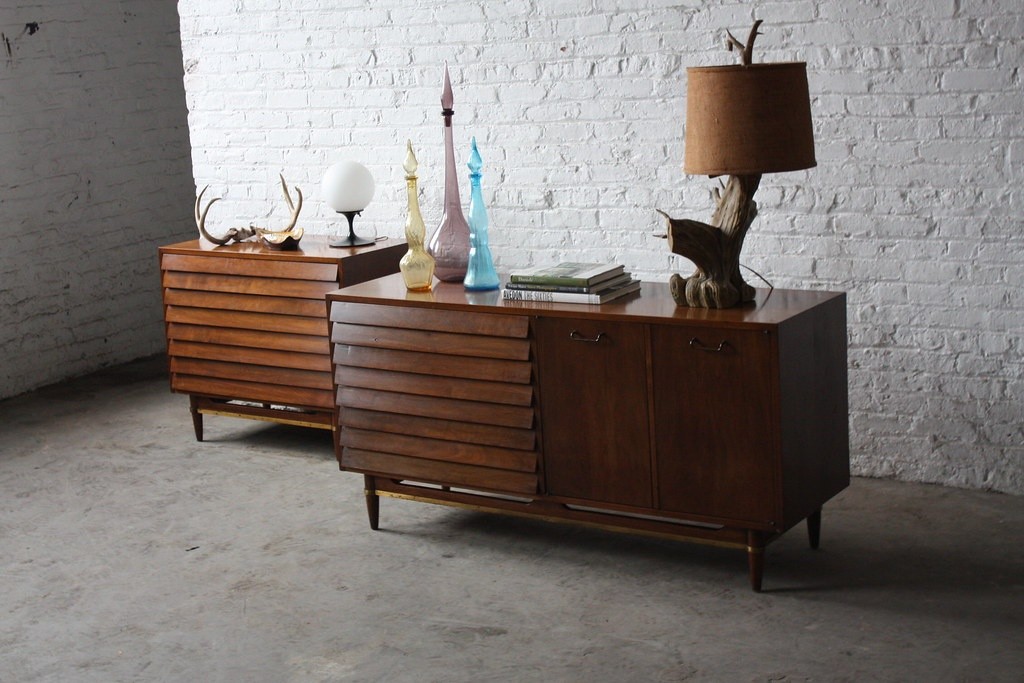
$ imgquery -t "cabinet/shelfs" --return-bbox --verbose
[325,271,851,592]
[157,231,409,443]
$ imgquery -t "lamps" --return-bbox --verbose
[652,18,817,309]
[326,161,376,249]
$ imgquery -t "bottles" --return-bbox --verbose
[427,59,470,282]
[399,139,436,291]
[464,136,500,291]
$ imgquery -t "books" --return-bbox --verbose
[510,261,625,287]
[505,271,632,293]
[501,279,642,305]
